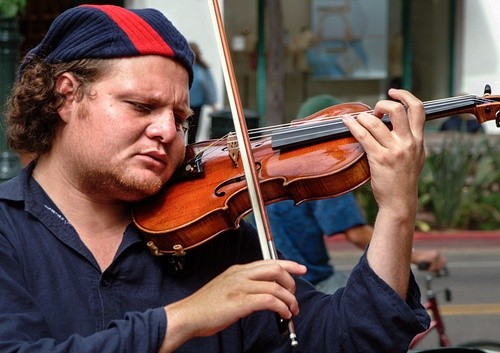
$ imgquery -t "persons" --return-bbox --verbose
[246,95,447,295]
[0,4,426,352]
[185,43,219,143]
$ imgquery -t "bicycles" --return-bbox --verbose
[405,256,500,353]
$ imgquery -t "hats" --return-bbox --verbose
[295,96,341,119]
[19,5,196,90]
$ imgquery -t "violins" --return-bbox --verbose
[131,83,500,258]
